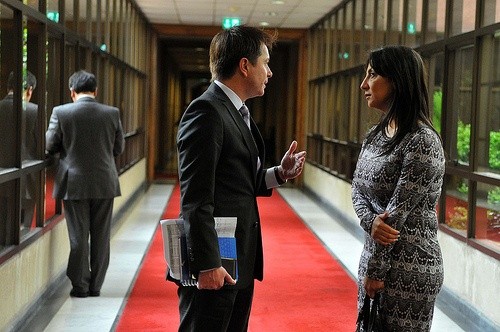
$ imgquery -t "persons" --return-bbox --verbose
[46,70,125,298]
[166,25,307,332]
[352,46,445,332]
[0,70,38,227]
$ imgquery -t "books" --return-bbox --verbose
[160,217,238,286]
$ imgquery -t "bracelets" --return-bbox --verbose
[274,165,287,185]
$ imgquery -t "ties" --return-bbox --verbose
[239,104,251,130]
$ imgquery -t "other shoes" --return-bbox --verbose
[89,290,100,296]
[70,288,88,297]
